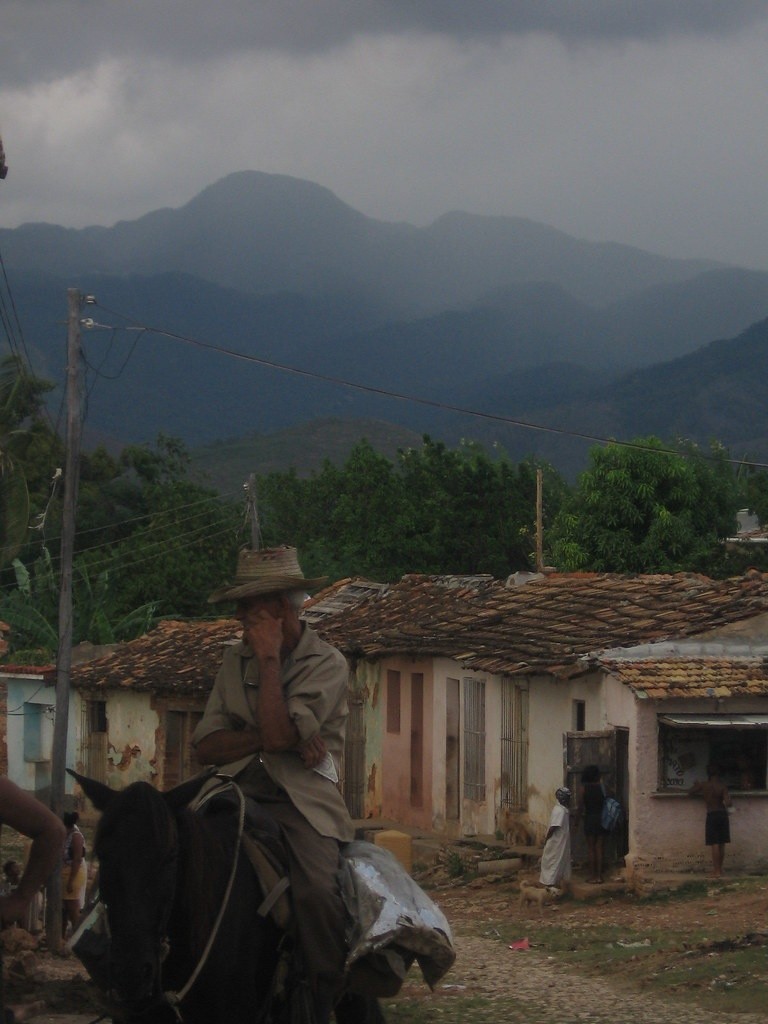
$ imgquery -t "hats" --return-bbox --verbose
[208,545,328,605]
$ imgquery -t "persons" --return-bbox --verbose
[63,812,86,937]
[539,788,574,901]
[2,861,23,898]
[194,545,351,1023]
[0,772,67,929]
[574,767,612,883]
[690,765,732,879]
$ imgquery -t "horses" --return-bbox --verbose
[63,763,385,1024]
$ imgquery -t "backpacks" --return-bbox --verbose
[599,778,625,831]
